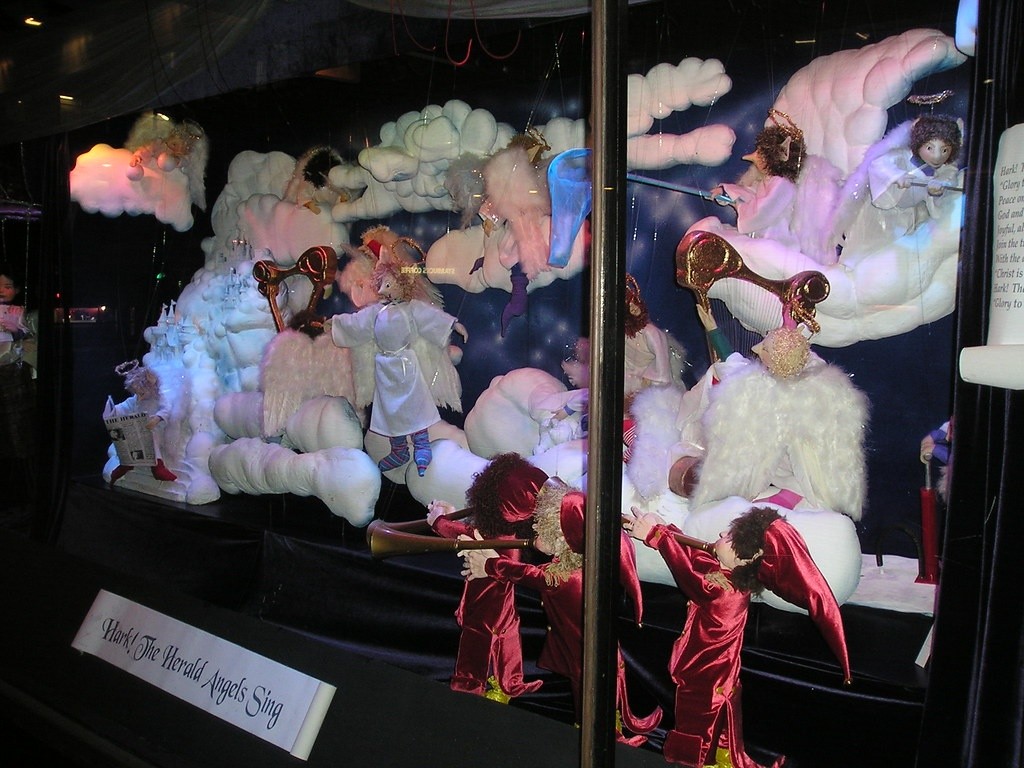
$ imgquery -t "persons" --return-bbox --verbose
[460,477,662,745]
[922,417,951,499]
[427,452,549,702]
[824,115,961,277]
[705,110,808,250]
[130,118,203,168]
[294,232,396,339]
[678,302,811,452]
[0,270,35,368]
[623,505,852,768]
[538,274,672,461]
[323,238,468,477]
[443,123,551,337]
[110,360,178,488]
